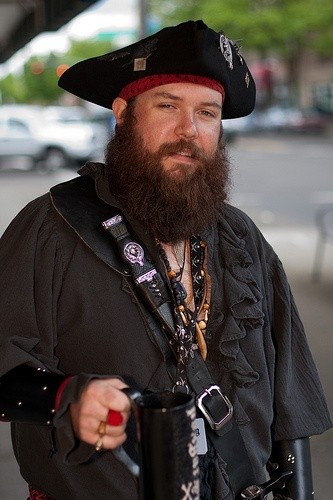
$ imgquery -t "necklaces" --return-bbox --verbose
[152,228,211,392]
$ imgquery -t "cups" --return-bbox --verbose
[109,386,201,500]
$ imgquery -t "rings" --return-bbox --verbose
[98,420,106,436]
[95,435,102,453]
[105,409,122,427]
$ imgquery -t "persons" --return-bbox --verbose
[0,20,333,500]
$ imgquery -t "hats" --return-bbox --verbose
[58,19,256,120]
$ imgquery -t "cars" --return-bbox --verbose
[1,100,327,174]
